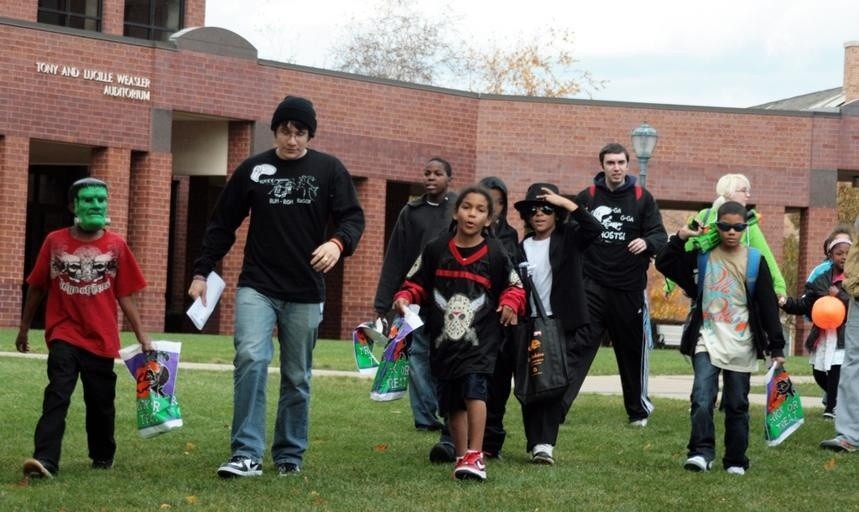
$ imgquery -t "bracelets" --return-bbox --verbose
[191,275,209,282]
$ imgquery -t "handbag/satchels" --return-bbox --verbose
[117,340,184,440]
[369,301,425,402]
[761,360,805,446]
[511,317,570,407]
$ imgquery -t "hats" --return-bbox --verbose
[514,182,567,226]
[270,95,317,132]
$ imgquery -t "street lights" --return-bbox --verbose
[629,120,660,189]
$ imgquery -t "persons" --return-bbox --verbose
[15,176,151,479]
[187,96,365,479]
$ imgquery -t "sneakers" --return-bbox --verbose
[630,417,648,428]
[528,443,556,466]
[451,450,488,484]
[275,463,301,477]
[415,424,441,432]
[683,455,714,473]
[482,449,503,461]
[428,434,458,464]
[91,453,116,470]
[822,412,834,422]
[727,466,745,476]
[23,458,58,482]
[819,436,859,453]
[216,456,264,479]
